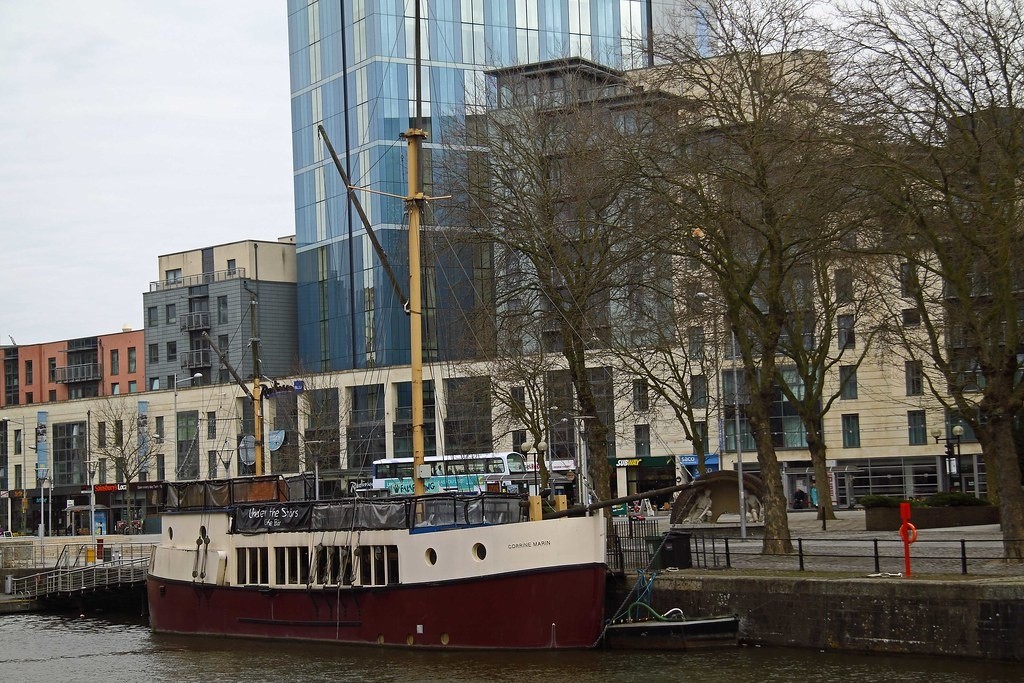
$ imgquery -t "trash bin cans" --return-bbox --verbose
[87,548,95,563]
[104,548,112,562]
[5,575,13,594]
[37,524,45,537]
[663,531,693,569]
[644,536,663,569]
[114,549,119,561]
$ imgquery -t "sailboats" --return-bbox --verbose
[145,129,609,652]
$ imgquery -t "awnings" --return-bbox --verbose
[587,456,676,468]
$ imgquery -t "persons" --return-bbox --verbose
[792,488,810,512]
[436,465,443,475]
[631,492,640,507]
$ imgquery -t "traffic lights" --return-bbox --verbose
[944,443,955,456]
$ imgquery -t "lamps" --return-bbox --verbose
[676,476,681,482]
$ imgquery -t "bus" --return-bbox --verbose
[371,452,530,500]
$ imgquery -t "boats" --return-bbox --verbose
[604,611,744,653]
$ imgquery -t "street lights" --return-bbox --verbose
[151,434,177,482]
[215,450,236,479]
[519,442,548,499]
[550,406,584,507]
[548,417,568,503]
[28,445,53,538]
[3,416,26,533]
[697,292,747,543]
[174,372,203,477]
[304,440,326,502]
[84,460,100,535]
[33,467,50,537]
[933,426,964,495]
